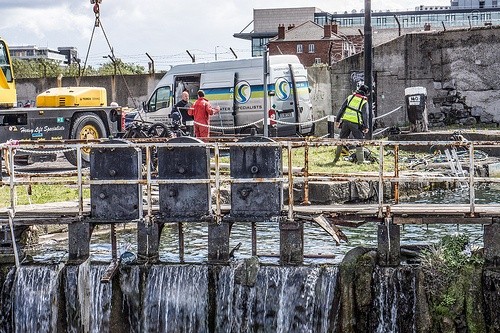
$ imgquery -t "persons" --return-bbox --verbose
[335,85,370,164]
[173,91,195,137]
[188,90,220,143]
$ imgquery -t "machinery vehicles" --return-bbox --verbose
[0,0,125,167]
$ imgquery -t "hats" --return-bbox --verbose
[359,84,370,96]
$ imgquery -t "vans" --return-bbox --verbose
[133,54,313,136]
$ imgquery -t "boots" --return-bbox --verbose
[356,147,370,165]
